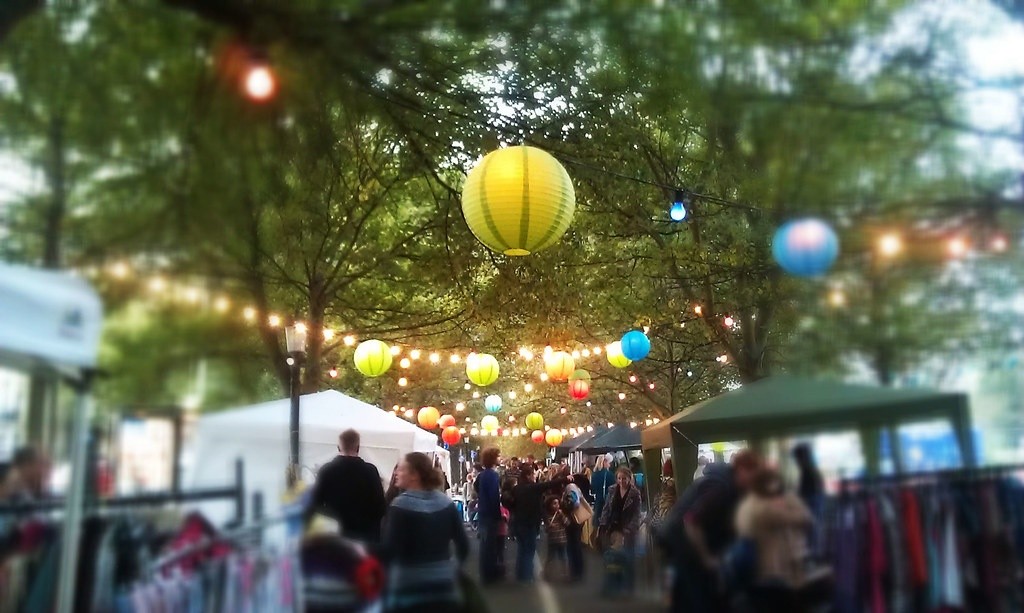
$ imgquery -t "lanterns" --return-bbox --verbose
[352,144,841,447]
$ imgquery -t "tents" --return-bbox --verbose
[639,371,981,515]
[0,258,453,613]
[557,425,647,473]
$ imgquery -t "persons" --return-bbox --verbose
[368,452,471,613]
[789,441,829,515]
[461,454,831,613]
[474,448,505,586]
[301,428,387,538]
[299,505,368,613]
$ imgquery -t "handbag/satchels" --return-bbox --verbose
[570,488,594,526]
